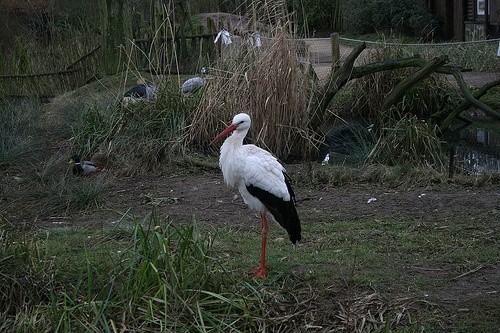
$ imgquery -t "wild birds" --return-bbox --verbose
[209,113,301,279]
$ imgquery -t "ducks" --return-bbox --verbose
[69,149,108,177]
